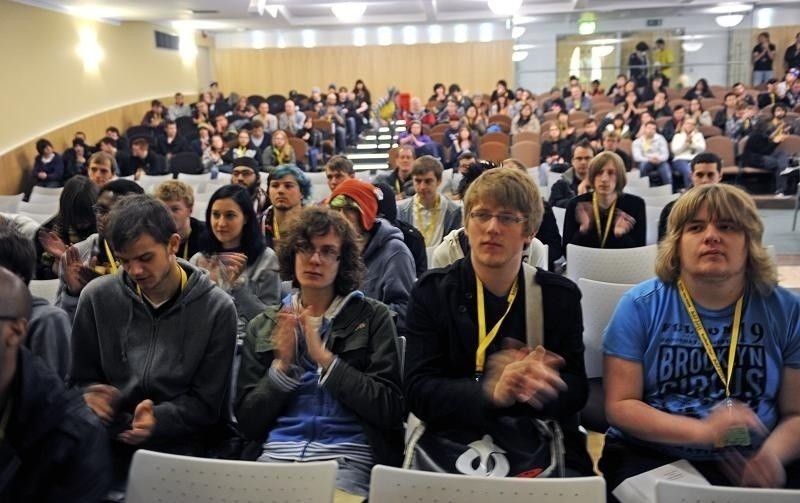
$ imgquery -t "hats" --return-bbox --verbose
[327,179,378,230]
[789,67,799,76]
[234,157,260,175]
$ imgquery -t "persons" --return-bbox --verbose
[59,140,92,185]
[598,184,799,502]
[371,183,429,281]
[656,153,724,245]
[397,80,542,160]
[167,92,193,120]
[0,267,114,502]
[154,119,190,159]
[141,99,171,127]
[187,185,281,391]
[126,138,171,178]
[106,125,133,152]
[543,73,799,140]
[55,178,146,324]
[71,195,237,502]
[323,179,417,339]
[556,117,742,147]
[99,137,141,177]
[230,158,275,252]
[743,116,800,200]
[85,151,115,189]
[75,128,98,159]
[629,43,649,84]
[562,151,647,257]
[594,133,633,174]
[193,77,371,172]
[403,166,594,478]
[233,205,403,503]
[23,138,66,199]
[651,38,676,86]
[785,33,799,72]
[670,119,707,193]
[430,164,550,271]
[542,123,573,172]
[255,164,311,258]
[547,139,596,208]
[632,120,673,186]
[749,31,776,86]
[396,155,462,249]
[155,182,217,258]
[0,233,49,320]
[31,176,99,281]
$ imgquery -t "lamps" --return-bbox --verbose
[578,11,597,35]
[709,2,752,27]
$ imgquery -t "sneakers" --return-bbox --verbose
[776,192,792,199]
[780,167,800,176]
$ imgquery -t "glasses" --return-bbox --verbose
[470,213,526,227]
[297,244,340,263]
[1,316,17,324]
[92,204,108,216]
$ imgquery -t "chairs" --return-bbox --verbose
[432,124,450,134]
[128,447,337,503]
[623,168,641,178]
[29,194,61,212]
[371,464,607,502]
[304,111,316,121]
[547,173,563,191]
[29,281,61,305]
[665,88,689,98]
[178,172,211,193]
[655,480,800,503]
[421,127,431,136]
[544,111,558,122]
[646,207,664,246]
[589,96,611,103]
[591,103,615,112]
[568,120,588,128]
[485,122,510,133]
[566,244,660,296]
[625,177,651,190]
[511,142,541,169]
[217,172,232,184]
[744,89,763,104]
[0,193,26,213]
[190,183,229,222]
[16,201,59,214]
[655,117,673,128]
[428,134,447,145]
[17,212,54,223]
[706,106,725,121]
[580,278,640,378]
[642,193,680,207]
[667,99,690,117]
[478,142,506,164]
[700,98,724,110]
[697,125,723,138]
[705,136,741,187]
[314,120,336,156]
[245,96,265,108]
[288,138,310,171]
[738,135,776,194]
[481,133,509,146]
[762,103,775,113]
[567,112,588,119]
[511,131,539,146]
[259,173,271,191]
[127,126,153,138]
[540,120,560,133]
[299,172,327,184]
[488,115,511,133]
[620,139,636,165]
[774,134,799,193]
[265,95,286,113]
[528,167,540,186]
[626,184,674,201]
[139,172,173,191]
[166,153,203,177]
[710,85,730,99]
[33,185,64,201]
[307,185,332,200]
[594,110,613,125]
